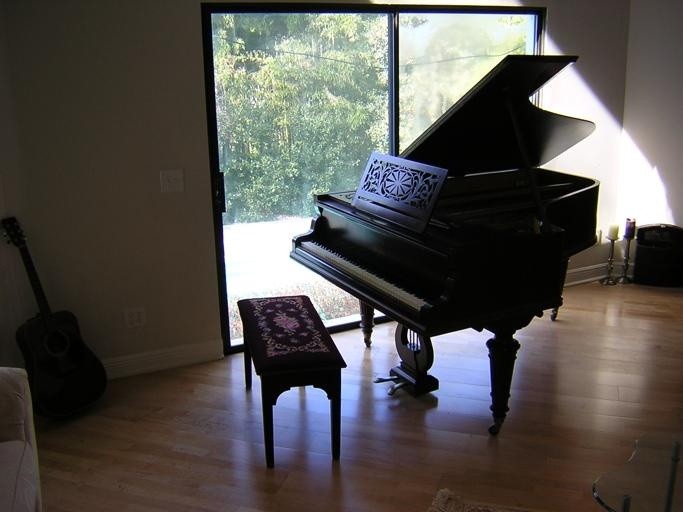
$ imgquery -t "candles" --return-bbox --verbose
[607,226,618,240]
[624,219,635,238]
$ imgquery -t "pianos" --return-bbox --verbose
[289,53,600,435]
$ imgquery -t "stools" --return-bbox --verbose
[236,295,348,470]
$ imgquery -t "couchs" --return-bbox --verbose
[0,365,44,512]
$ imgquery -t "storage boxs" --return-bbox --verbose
[634,223,683,288]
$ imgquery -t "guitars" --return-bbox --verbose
[0,216,109,425]
